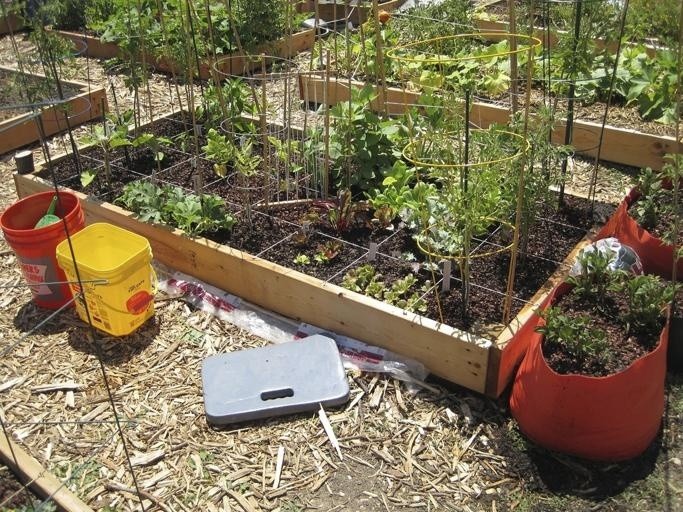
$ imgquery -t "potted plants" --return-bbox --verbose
[508,165,683,462]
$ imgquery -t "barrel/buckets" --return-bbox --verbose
[0,191,83,310]
[55,221,157,338]
